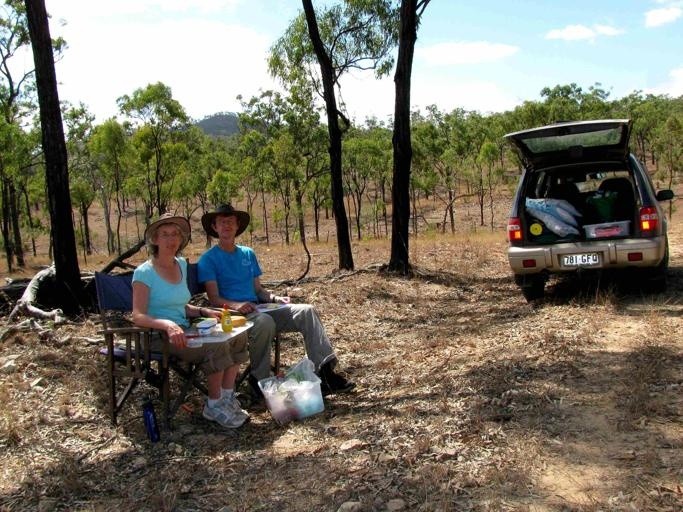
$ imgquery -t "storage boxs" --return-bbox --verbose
[582,221,630,238]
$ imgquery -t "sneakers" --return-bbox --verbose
[321,375,356,392]
[203,397,250,428]
[248,375,262,396]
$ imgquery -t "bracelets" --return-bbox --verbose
[198,306,203,317]
[272,294,277,303]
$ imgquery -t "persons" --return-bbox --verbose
[197,205,354,407]
[131,212,249,429]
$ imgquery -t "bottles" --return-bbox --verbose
[141,400,161,442]
[222,303,232,333]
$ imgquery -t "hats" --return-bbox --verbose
[144,213,191,250]
[201,205,250,238]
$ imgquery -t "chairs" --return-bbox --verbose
[95,272,209,430]
[257,372,325,425]
[186,258,280,392]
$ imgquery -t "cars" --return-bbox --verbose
[503,117,673,298]
